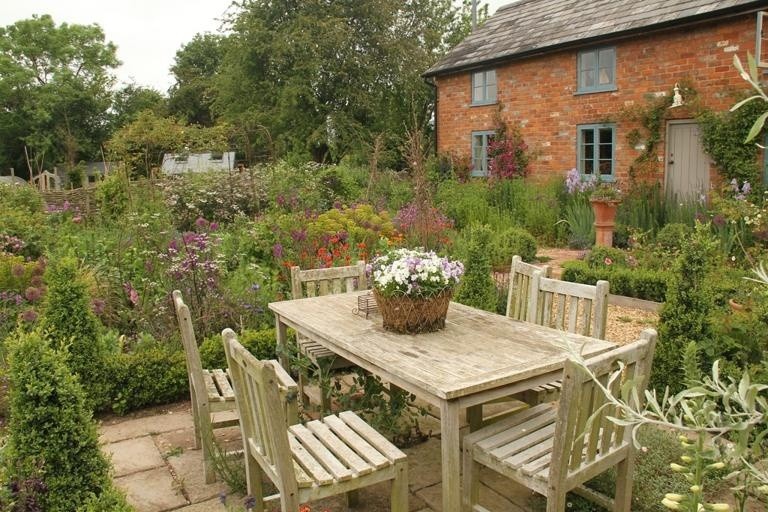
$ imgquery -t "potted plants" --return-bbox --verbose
[587,185,623,225]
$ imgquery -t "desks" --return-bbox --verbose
[266,287,621,510]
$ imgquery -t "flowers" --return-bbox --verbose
[366,246,463,298]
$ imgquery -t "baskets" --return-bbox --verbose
[372,288,454,334]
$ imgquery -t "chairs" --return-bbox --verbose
[171,287,302,487]
[467,269,611,471]
[502,256,553,326]
[290,259,389,414]
[218,328,413,512]
[458,326,657,512]
